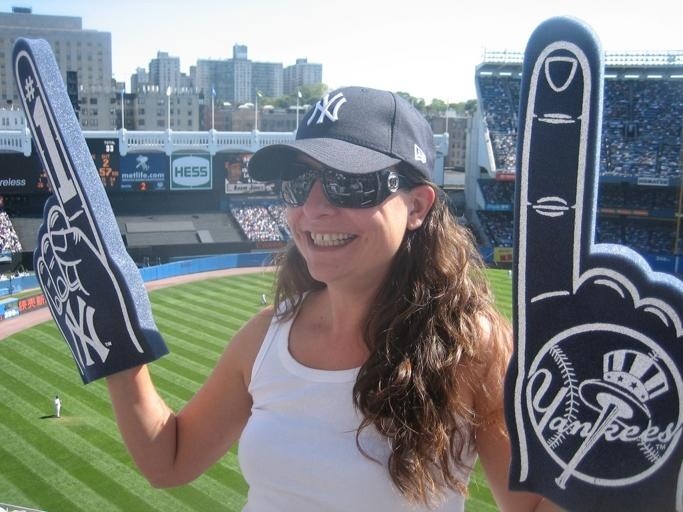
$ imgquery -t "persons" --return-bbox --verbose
[0,195,21,253]
[225,152,246,190]
[102,84,569,512]
[232,203,291,241]
[476,68,682,260]
[54,395,62,418]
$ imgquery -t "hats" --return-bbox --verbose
[248,87,435,183]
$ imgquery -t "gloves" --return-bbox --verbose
[13,37,170,387]
[501,14,683,510]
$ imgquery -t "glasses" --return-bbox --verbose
[277,159,410,208]
